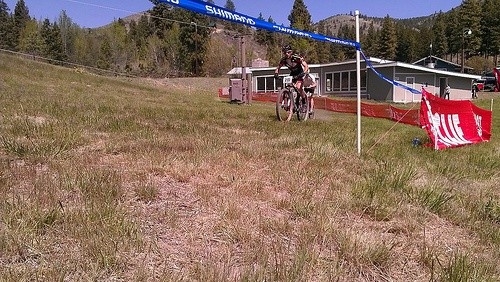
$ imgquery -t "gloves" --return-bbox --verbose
[274,73,279,79]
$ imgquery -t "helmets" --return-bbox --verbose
[283,44,293,52]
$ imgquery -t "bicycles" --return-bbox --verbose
[272,72,316,123]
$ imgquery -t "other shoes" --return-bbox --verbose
[302,94,308,107]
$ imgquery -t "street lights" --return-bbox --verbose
[461,26,472,72]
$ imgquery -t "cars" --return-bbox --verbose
[484,78,499,92]
[476,79,486,90]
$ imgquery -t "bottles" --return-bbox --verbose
[411,136,420,147]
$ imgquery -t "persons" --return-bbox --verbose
[274,44,317,115]
[444,84,451,100]
[472,83,481,99]
